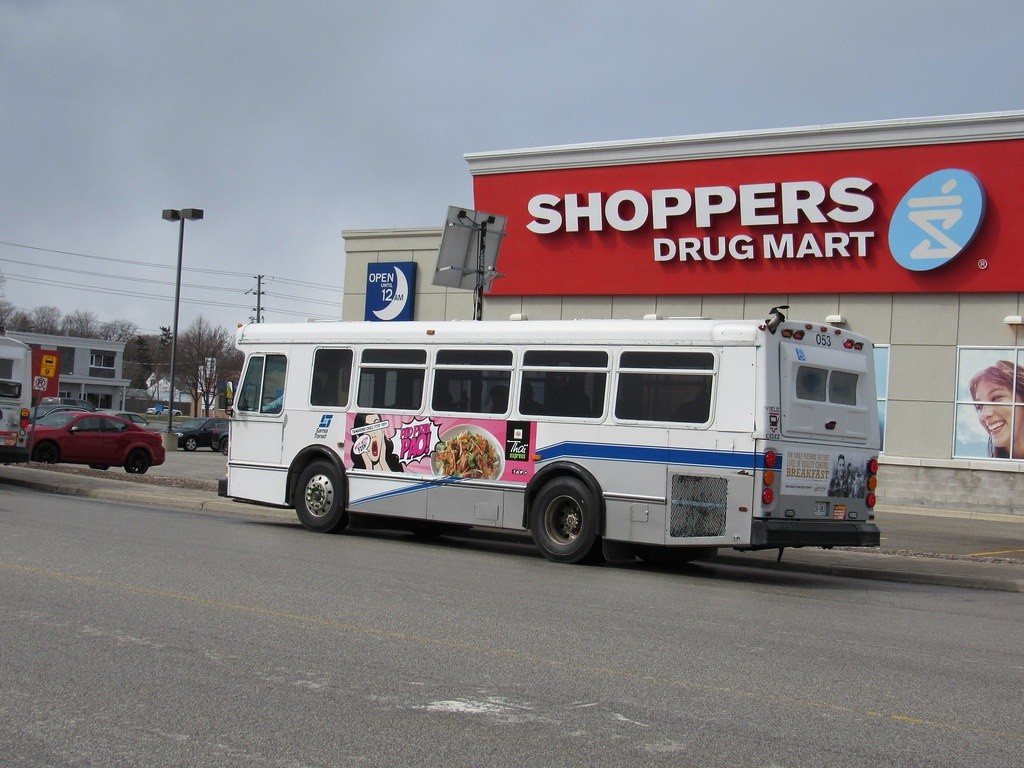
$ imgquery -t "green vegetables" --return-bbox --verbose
[437,430,492,478]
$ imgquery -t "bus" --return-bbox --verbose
[217,307,884,567]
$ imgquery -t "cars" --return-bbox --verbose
[25,411,166,474]
[212,421,230,456]
[28,405,153,434]
[164,416,227,453]
[146,405,183,417]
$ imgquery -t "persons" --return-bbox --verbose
[970,360,1024,459]
[253,377,711,422]
[489,386,509,414]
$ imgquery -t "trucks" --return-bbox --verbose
[0,337,33,457]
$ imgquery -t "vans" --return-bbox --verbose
[40,397,97,413]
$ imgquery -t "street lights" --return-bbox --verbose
[160,208,203,455]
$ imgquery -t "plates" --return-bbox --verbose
[430,424,505,481]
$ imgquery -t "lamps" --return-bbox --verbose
[1003,315,1024,324]
[825,315,845,325]
